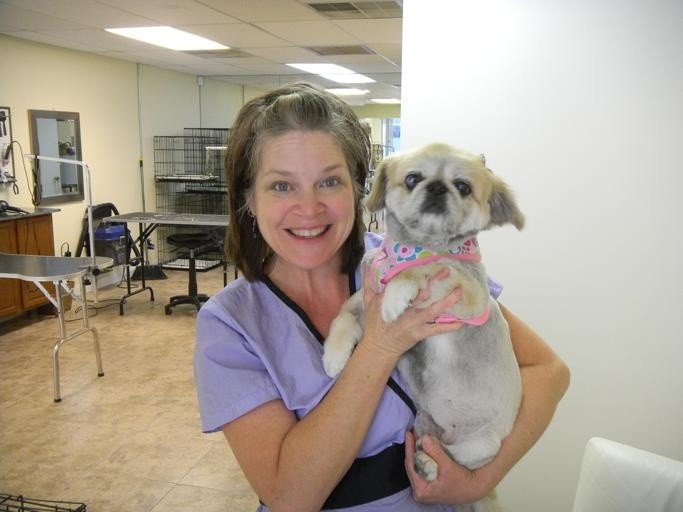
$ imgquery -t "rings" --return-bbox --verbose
[407,301,414,308]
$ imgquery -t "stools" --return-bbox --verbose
[164,233,213,316]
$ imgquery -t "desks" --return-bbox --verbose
[102,210,238,315]
[0,253,114,403]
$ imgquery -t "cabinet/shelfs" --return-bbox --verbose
[0,207,61,325]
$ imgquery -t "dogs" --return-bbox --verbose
[318,139,528,512]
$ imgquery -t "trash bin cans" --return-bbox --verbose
[83,205,128,265]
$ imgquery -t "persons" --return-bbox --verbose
[191,80,571,512]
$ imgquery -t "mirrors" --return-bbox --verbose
[28,108,85,206]
